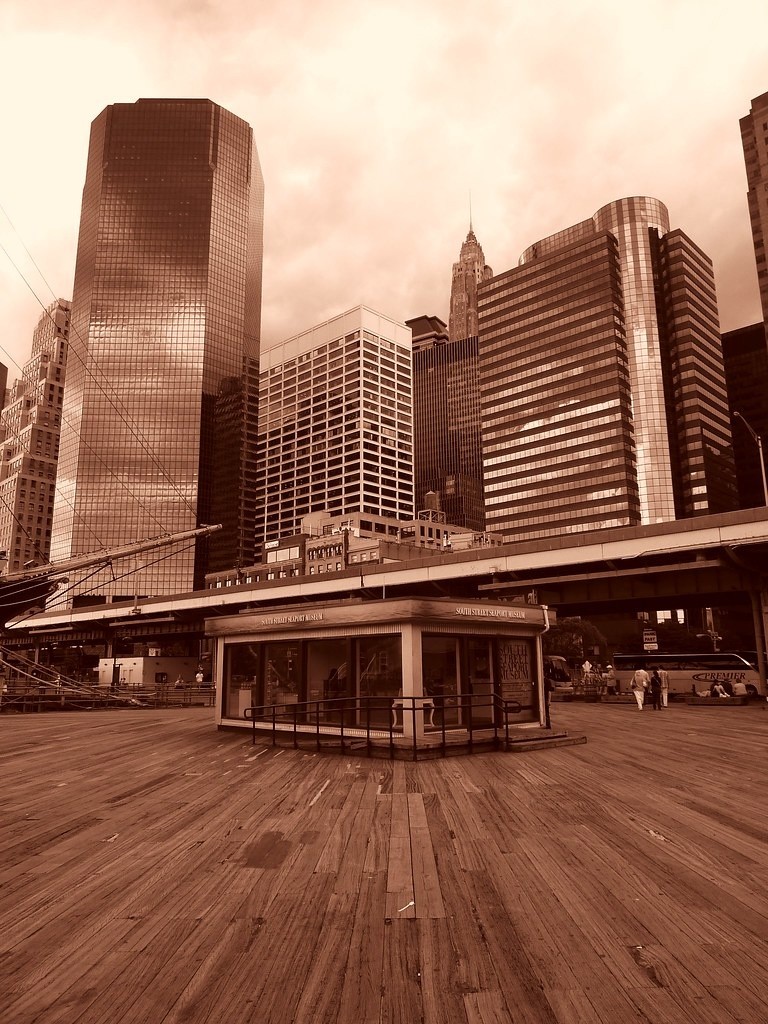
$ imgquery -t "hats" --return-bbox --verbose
[606,665,613,668]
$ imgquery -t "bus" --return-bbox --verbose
[612,650,768,697]
[543,655,575,697]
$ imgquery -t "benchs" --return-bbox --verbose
[688,697,748,705]
[600,694,655,704]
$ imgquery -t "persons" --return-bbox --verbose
[651,671,661,710]
[119,678,126,686]
[630,665,651,710]
[195,670,203,688]
[175,679,187,688]
[543,670,551,728]
[657,667,669,708]
[605,665,617,696]
[711,677,734,698]
[734,678,747,696]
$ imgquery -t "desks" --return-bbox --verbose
[391,698,436,727]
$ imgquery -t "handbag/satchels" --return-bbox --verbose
[632,676,636,689]
[642,682,648,688]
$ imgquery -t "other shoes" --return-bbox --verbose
[719,693,726,697]
[724,693,730,697]
[545,726,551,729]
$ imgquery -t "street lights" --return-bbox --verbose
[696,629,722,652]
[111,632,132,696]
[41,639,58,679]
[734,411,767,507]
[132,500,178,613]
